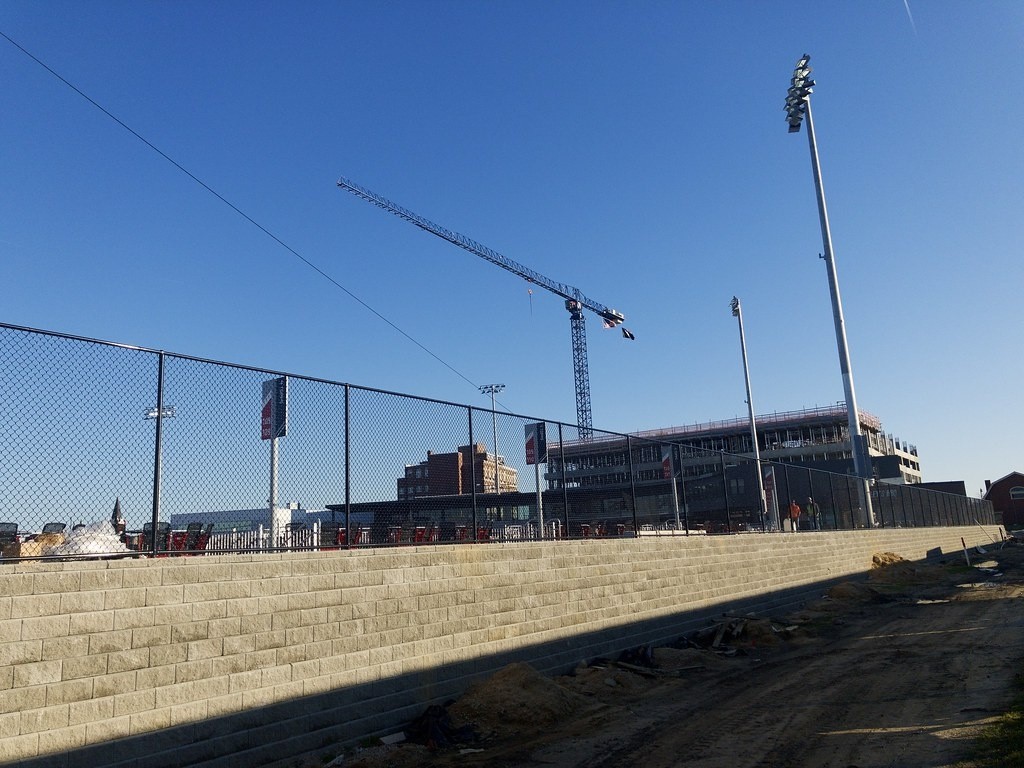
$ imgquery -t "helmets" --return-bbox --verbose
[791,499,796,503]
[806,497,812,503]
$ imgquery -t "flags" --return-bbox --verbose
[603,318,616,328]
[622,327,635,340]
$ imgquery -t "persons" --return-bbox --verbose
[807,498,820,530]
[787,500,802,531]
